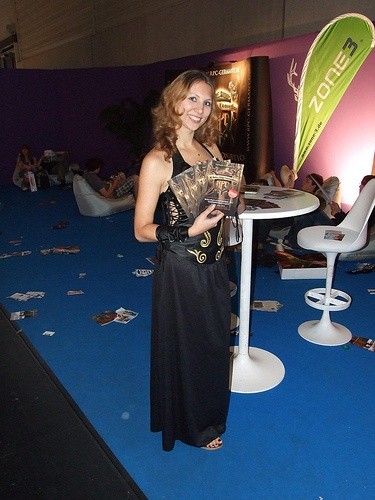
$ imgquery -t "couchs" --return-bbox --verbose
[73,174,135,217]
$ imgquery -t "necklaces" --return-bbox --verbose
[195,151,201,156]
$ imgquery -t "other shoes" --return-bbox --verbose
[276,238,294,251]
[201,437,223,450]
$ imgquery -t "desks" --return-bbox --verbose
[225,186,320,394]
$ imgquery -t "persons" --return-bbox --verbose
[12,146,71,190]
[253,170,323,262]
[278,175,375,250]
[134,70,246,452]
[84,160,139,202]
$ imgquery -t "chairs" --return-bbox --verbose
[296,178,375,347]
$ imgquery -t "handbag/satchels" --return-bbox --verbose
[222,212,242,246]
[40,170,50,189]
